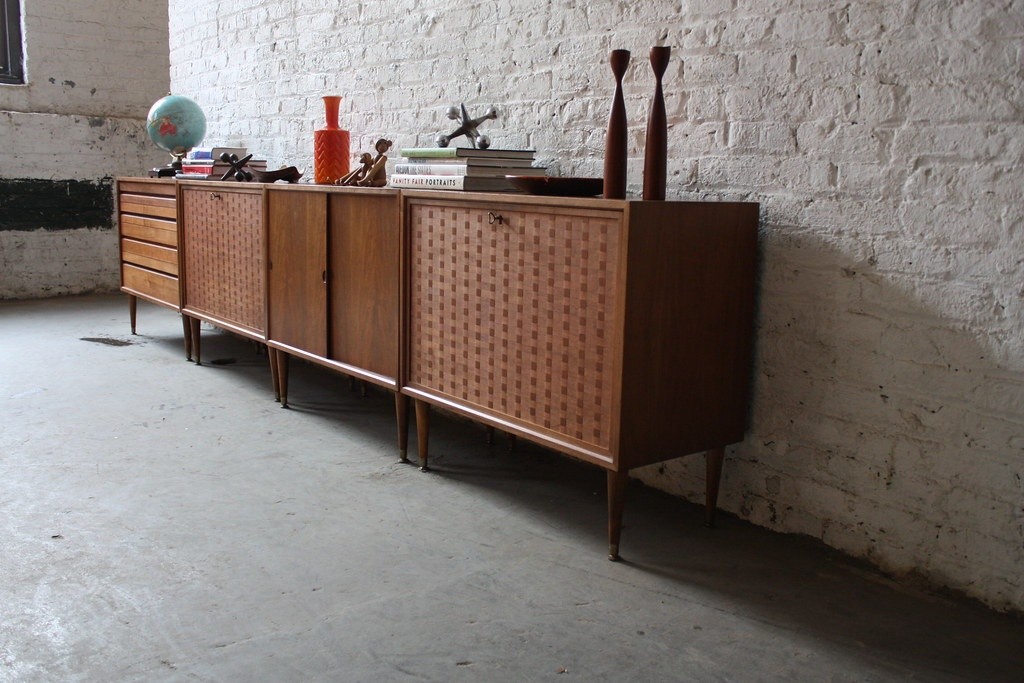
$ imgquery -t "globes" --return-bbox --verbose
[146,91,206,178]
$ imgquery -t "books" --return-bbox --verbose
[390,147,549,191]
[175,147,267,182]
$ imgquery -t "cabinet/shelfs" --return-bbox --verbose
[115,176,262,360]
[265,183,495,464]
[178,179,355,402]
[399,189,761,562]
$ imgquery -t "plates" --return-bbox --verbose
[247,164,307,183]
[504,174,605,196]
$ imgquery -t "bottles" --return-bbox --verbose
[312,95,351,184]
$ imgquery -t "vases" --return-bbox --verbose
[643,46,671,199]
[314,96,351,186]
[602,49,631,198]
